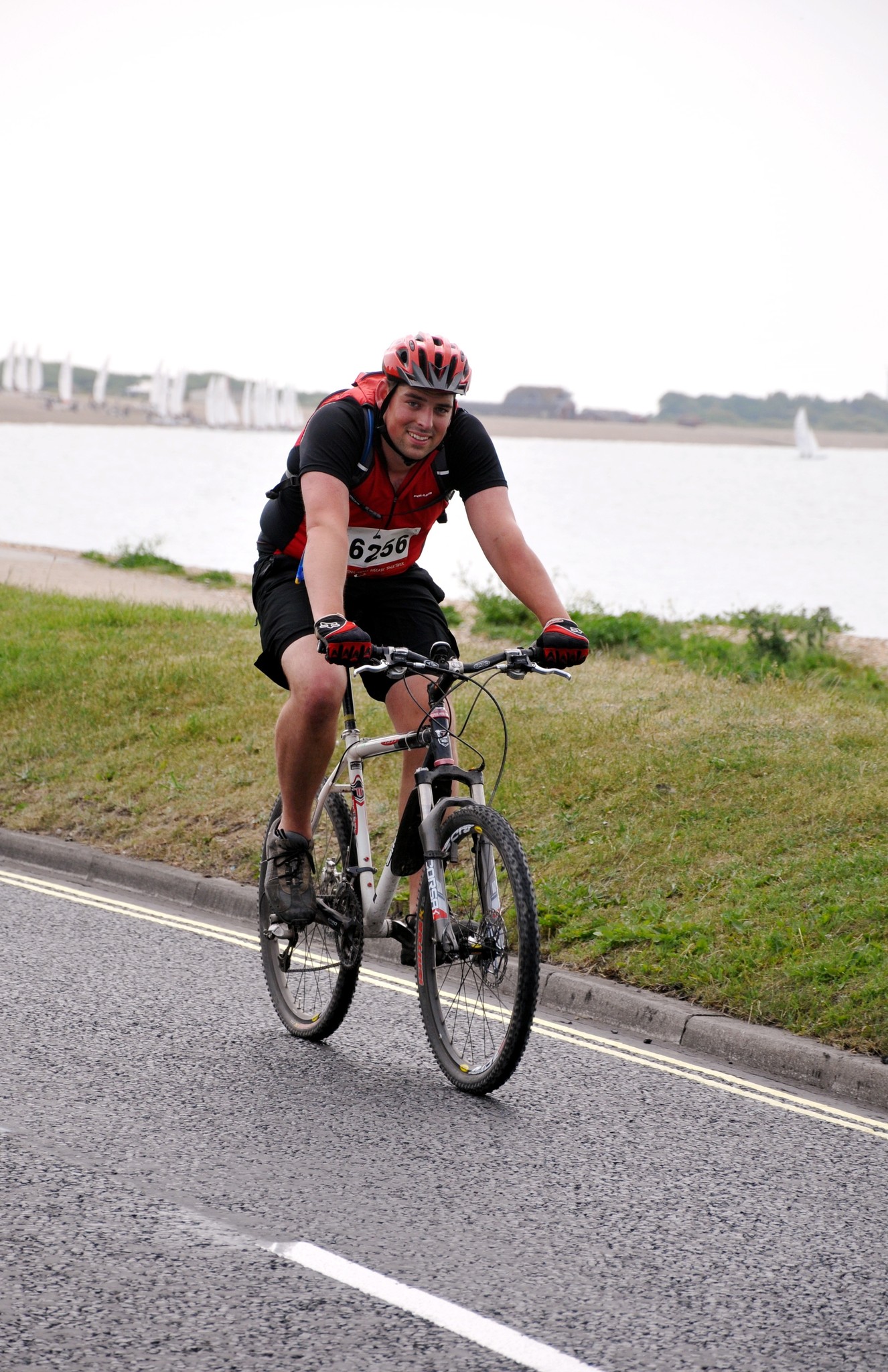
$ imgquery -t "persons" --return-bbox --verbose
[252,334,589,965]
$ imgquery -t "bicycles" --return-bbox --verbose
[255,642,574,1104]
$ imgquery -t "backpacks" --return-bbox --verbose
[266,371,386,502]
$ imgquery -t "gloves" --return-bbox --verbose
[530,617,589,669]
[313,612,372,669]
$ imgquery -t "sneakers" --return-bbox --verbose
[264,815,317,925]
[399,919,484,967]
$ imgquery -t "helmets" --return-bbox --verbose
[382,331,472,396]
